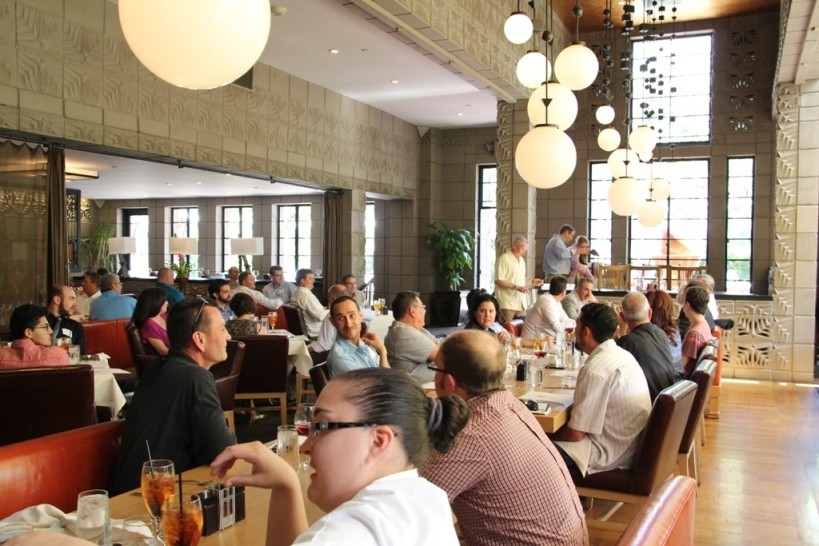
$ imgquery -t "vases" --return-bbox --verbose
[177,277,187,295]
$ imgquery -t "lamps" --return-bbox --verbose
[231,238,264,274]
[168,238,198,255]
[505,0,677,226]
[117,0,270,91]
[108,236,136,277]
[0,163,99,182]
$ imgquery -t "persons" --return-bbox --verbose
[1,263,718,546]
[494,224,590,324]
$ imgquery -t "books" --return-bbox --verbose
[525,401,551,415]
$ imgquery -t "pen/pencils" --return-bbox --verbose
[545,366,564,369]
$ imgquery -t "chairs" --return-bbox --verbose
[598,263,706,291]
[0,306,723,546]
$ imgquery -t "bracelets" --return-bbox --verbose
[513,285,517,289]
[532,279,534,284]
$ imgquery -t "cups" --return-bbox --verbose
[276,425,300,475]
[261,315,268,332]
[76,488,109,546]
[533,340,548,358]
[162,493,203,546]
[57,338,80,364]
[295,402,317,437]
[565,320,574,333]
[199,267,209,274]
[374,298,388,315]
[122,515,167,546]
[529,367,543,387]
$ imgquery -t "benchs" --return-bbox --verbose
[1,419,127,515]
[80,318,135,368]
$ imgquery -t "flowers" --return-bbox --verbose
[166,251,193,277]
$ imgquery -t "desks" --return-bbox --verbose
[424,340,589,433]
[267,328,313,378]
[75,351,126,420]
[62,432,327,546]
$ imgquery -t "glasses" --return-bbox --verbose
[409,305,426,311]
[192,294,209,334]
[307,419,399,439]
[29,324,49,331]
[428,362,456,378]
[111,283,123,286]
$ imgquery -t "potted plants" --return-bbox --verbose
[425,222,481,327]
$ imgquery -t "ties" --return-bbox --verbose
[51,318,61,345]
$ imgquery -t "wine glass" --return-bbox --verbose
[141,459,175,538]
[269,312,277,332]
[316,269,321,278]
[253,269,260,280]
